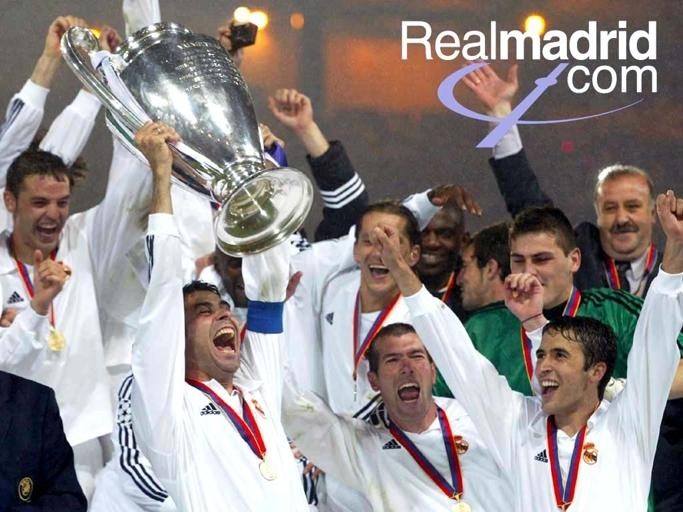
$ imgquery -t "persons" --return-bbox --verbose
[0,15,683,512]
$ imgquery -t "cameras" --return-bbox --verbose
[231,22,258,49]
